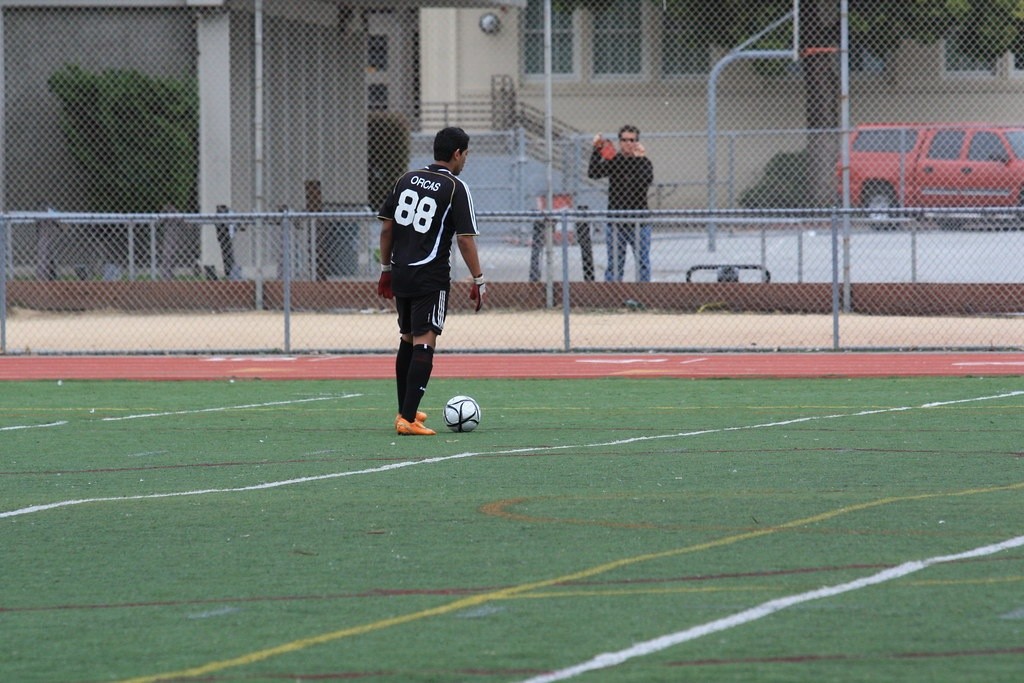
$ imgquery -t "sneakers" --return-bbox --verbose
[394,412,427,428]
[396,418,436,434]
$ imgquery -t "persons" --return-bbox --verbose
[587,125,654,282]
[376,127,487,435]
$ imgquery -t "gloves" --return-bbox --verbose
[469,273,487,312]
[377,264,395,299]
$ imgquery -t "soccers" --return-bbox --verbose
[443,395,482,434]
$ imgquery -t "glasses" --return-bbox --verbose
[619,138,638,142]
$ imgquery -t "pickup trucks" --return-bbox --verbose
[836,123,1024,233]
